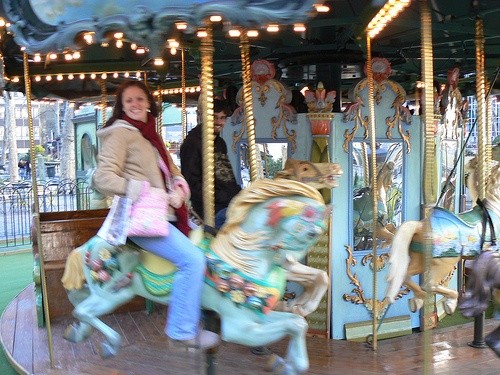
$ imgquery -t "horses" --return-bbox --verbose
[61,142,500,375]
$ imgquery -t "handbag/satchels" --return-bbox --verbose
[128,188,169,237]
[96,179,133,247]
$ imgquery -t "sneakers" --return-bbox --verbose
[175,330,220,349]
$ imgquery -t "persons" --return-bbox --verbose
[18,157,31,174]
[92,79,221,353]
[179,98,244,229]
